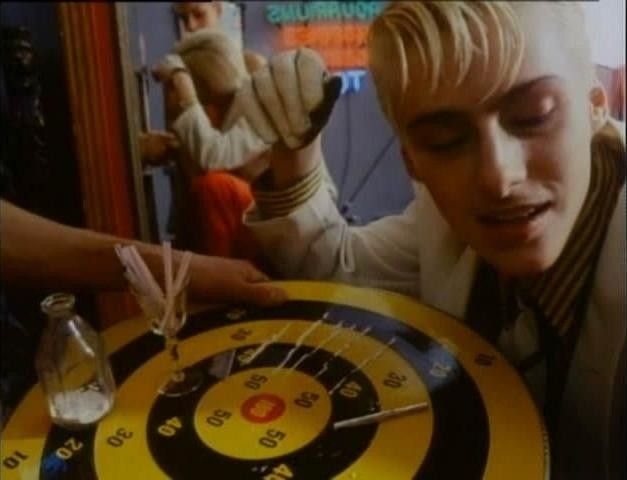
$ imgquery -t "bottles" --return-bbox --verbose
[40,290,117,428]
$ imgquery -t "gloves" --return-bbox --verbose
[150,55,189,85]
[240,47,342,151]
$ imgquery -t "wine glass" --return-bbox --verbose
[130,271,197,400]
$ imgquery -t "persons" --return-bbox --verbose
[234,1,627,479]
[1,196,287,312]
[138,28,338,259]
[161,0,269,126]
